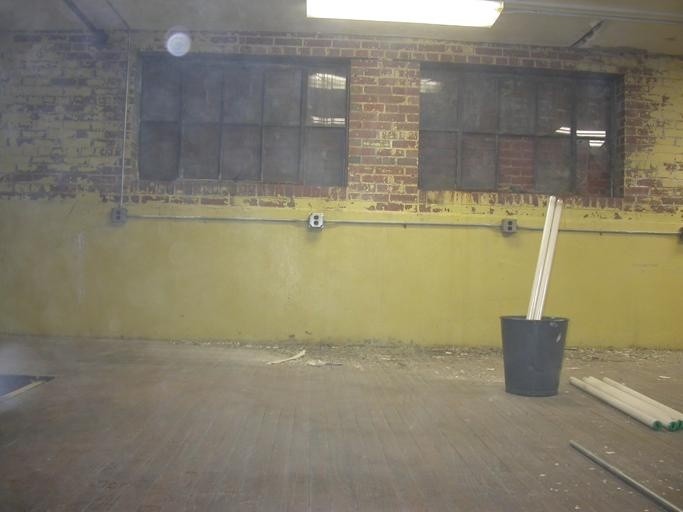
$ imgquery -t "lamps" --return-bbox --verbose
[306,0,505,29]
